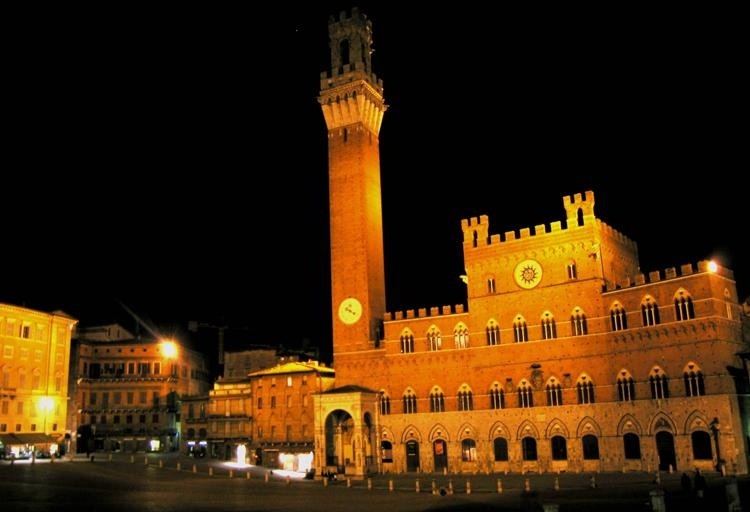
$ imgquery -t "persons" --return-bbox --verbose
[681,471,691,493]
[694,469,706,500]
